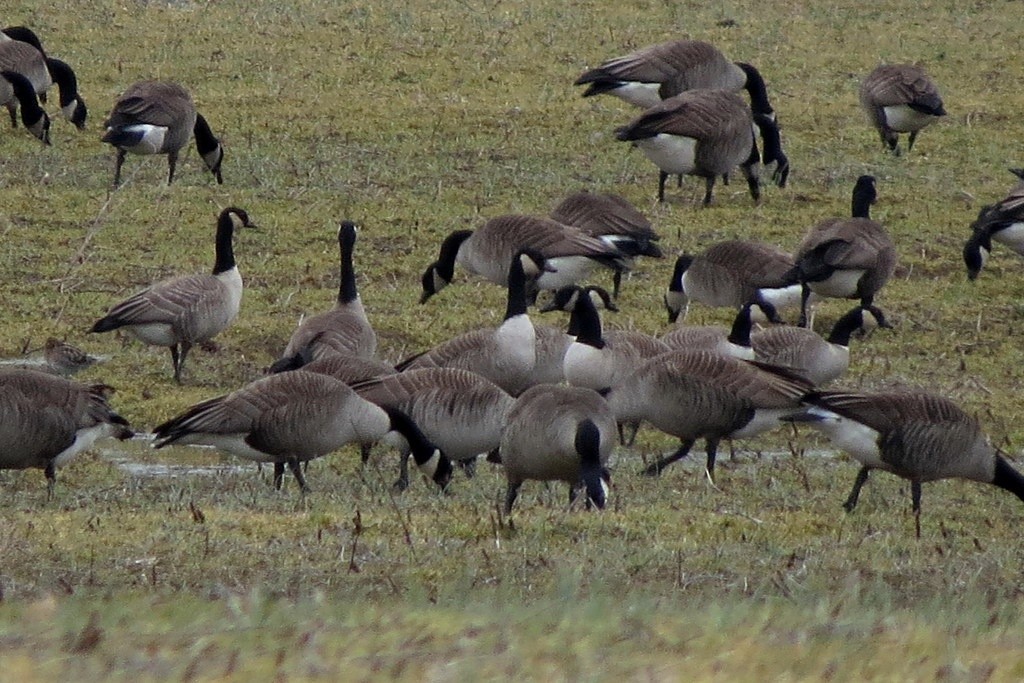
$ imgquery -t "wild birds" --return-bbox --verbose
[0,25,87,147]
[961,167,1024,284]
[100,79,224,187]
[84,206,259,381]
[161,165,892,519]
[858,64,946,157]
[572,41,789,209]
[777,387,1023,541]
[0,338,135,505]
